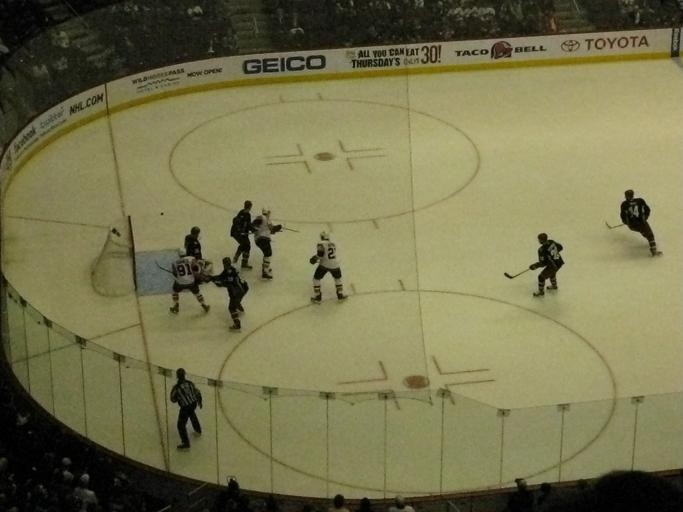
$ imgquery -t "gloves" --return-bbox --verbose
[529,262,540,270]
[310,257,318,265]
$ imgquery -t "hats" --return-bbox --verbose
[79,473,90,484]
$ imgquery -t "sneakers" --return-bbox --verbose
[227,324,241,330]
[176,444,191,449]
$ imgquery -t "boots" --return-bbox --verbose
[200,302,211,313]
[241,258,254,270]
[232,256,238,263]
[651,249,664,255]
[532,290,546,296]
[169,302,180,314]
[337,292,349,299]
[310,292,323,303]
[260,268,274,281]
[545,284,558,290]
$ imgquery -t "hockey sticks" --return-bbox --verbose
[605,221,625,229]
[154,259,173,274]
[504,269,530,279]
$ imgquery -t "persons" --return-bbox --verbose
[310,229,346,300]
[230,200,253,267]
[620,189,662,256]
[252,209,282,278]
[530,232,565,294]
[185,228,202,259]
[209,258,250,331]
[169,248,211,312]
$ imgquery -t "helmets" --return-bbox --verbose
[320,230,331,240]
[243,199,253,210]
[261,205,272,215]
[222,256,232,268]
[538,232,548,242]
[176,367,186,378]
[177,246,187,257]
[190,225,201,236]
[624,189,634,200]
